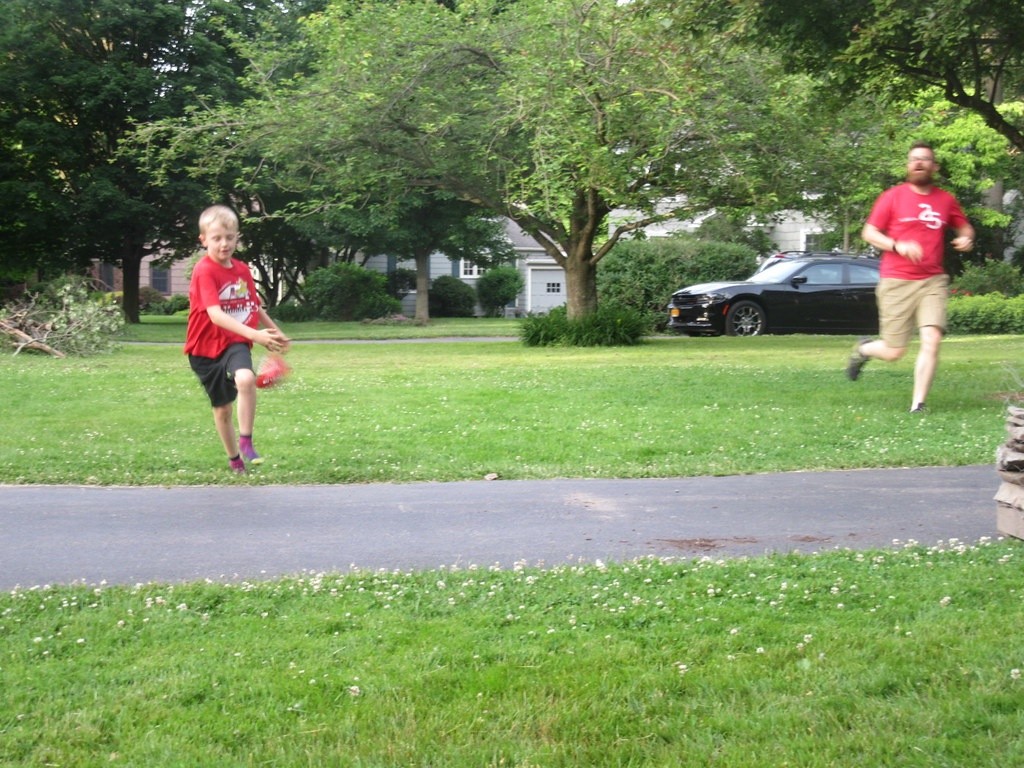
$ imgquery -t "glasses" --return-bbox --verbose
[909,157,933,162]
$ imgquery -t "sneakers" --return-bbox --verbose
[848,340,869,382]
[907,402,931,414]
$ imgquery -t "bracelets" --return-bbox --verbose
[892,241,898,255]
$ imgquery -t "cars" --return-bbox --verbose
[663,254,883,335]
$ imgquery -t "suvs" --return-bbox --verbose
[753,251,874,279]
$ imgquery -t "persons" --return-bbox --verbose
[847,141,975,412]
[183,205,290,474]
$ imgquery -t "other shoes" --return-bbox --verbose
[229,436,265,479]
